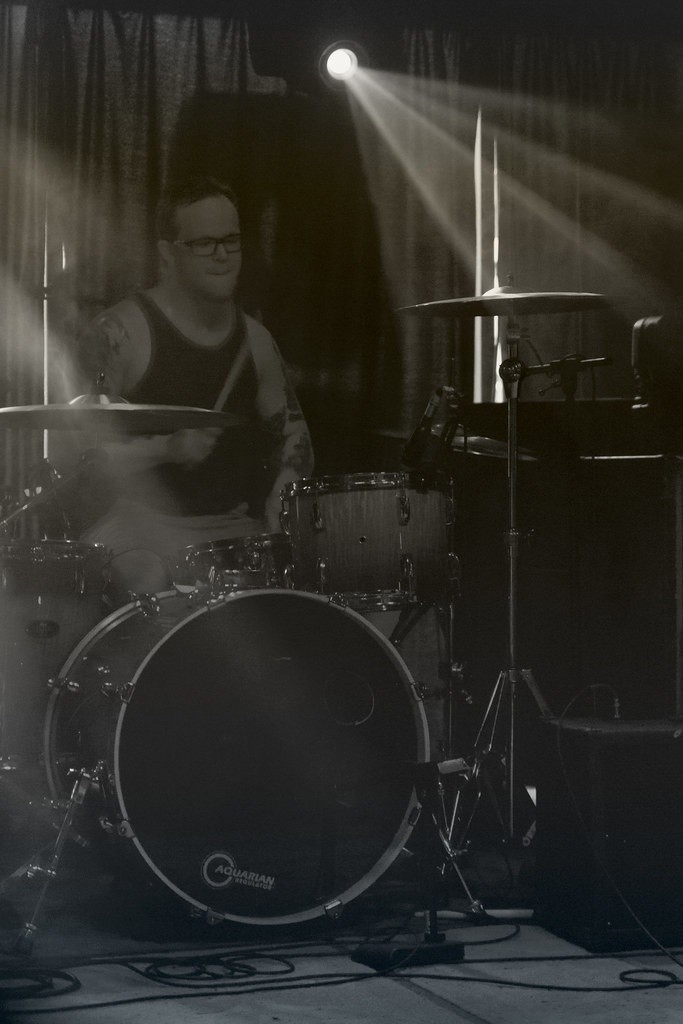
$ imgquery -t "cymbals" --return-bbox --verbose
[442,430,544,464]
[0,390,248,431]
[389,286,612,316]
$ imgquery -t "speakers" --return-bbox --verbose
[535,715,683,955]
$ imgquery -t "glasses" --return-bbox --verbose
[167,232,243,257]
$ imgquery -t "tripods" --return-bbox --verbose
[434,322,568,914]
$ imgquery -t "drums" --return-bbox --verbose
[167,531,293,591]
[40,583,435,930]
[0,535,117,779]
[280,473,461,607]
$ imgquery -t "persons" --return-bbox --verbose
[65,177,316,635]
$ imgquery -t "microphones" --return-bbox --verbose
[48,465,74,529]
[401,389,444,468]
